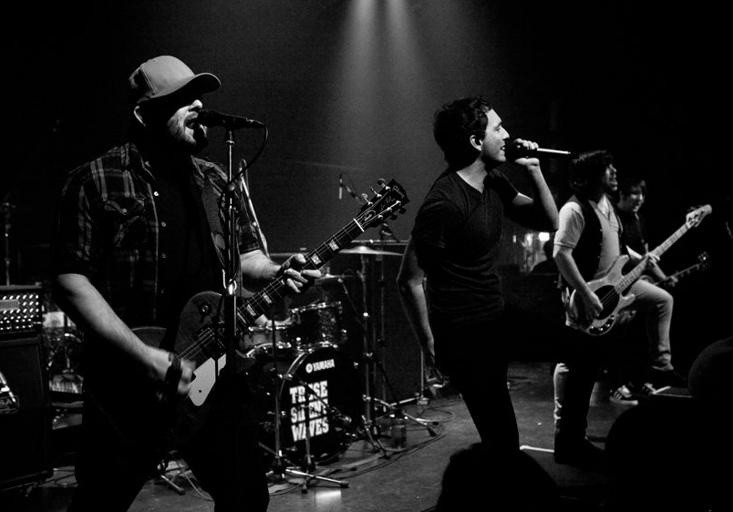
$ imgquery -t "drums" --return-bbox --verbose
[289,300,346,351]
[263,247,313,275]
[289,269,346,309]
[238,340,364,471]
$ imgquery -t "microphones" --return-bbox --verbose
[510,140,571,164]
[198,109,259,130]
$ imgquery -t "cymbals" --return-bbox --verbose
[331,244,406,258]
[350,235,410,250]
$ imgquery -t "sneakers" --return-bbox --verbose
[609,383,671,405]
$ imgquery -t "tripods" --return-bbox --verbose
[258,229,442,495]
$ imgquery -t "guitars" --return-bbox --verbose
[565,204,711,336]
[121,178,405,411]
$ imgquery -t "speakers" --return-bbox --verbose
[328,247,426,420]
[0,334,53,482]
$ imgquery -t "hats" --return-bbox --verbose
[128,55,223,106]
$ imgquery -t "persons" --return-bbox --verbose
[550,150,671,466]
[605,167,679,406]
[394,95,561,458]
[431,335,733,511]
[45,54,322,512]
